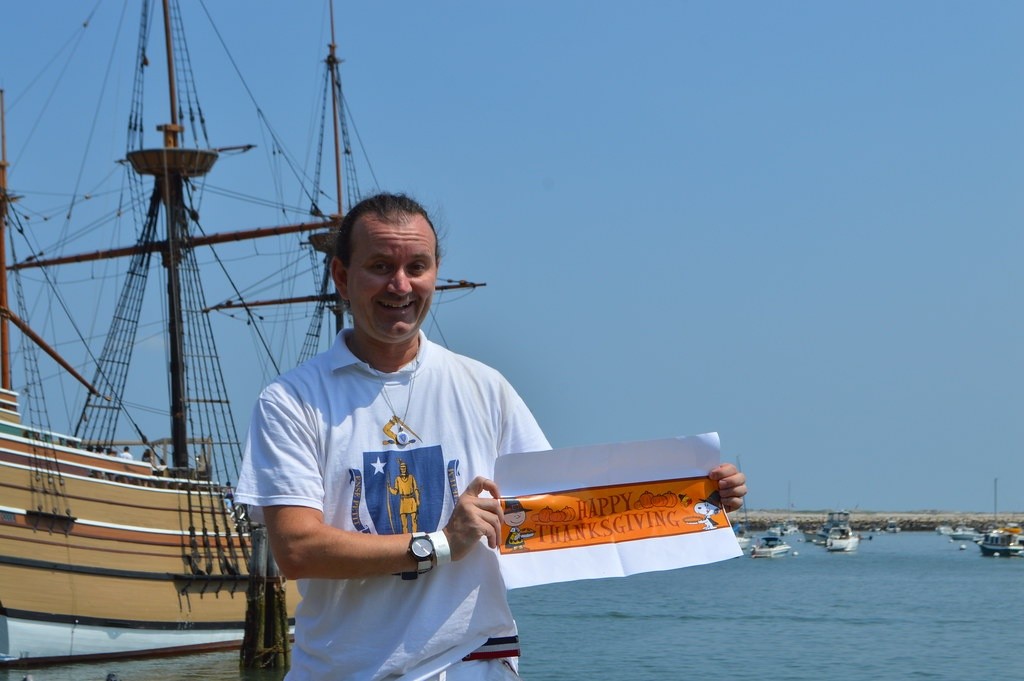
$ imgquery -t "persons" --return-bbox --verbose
[66,440,73,448]
[118,447,132,484]
[23,430,31,438]
[105,448,115,480]
[87,446,103,479]
[225,482,234,498]
[34,433,40,440]
[142,449,151,487]
[232,194,748,681]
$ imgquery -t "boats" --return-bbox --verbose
[733,521,759,550]
[803,510,850,545]
[936,526,954,535]
[979,522,1024,559]
[750,536,791,560]
[826,527,859,551]
[767,518,799,536]
[949,525,977,539]
[886,521,901,533]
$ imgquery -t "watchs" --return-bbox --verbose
[406,531,434,575]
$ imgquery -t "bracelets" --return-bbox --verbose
[427,530,452,567]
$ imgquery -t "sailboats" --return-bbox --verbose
[1,1,488,668]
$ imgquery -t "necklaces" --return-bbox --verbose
[368,353,418,445]
